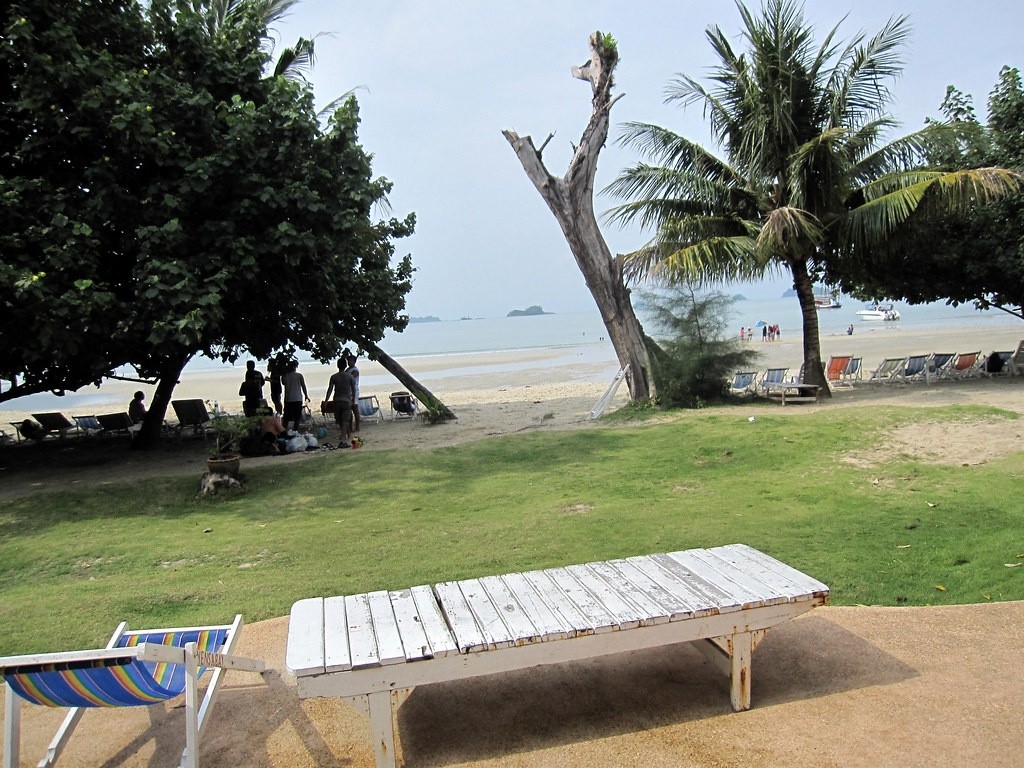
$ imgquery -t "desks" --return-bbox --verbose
[286,544,827,768]
[759,382,821,405]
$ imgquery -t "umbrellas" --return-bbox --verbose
[756,321,767,327]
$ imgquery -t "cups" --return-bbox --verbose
[352,438,359,449]
[318,424,326,438]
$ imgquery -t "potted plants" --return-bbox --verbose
[202,405,272,477]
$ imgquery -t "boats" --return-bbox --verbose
[856,304,901,321]
[814,298,842,308]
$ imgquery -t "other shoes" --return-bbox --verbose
[338,442,350,448]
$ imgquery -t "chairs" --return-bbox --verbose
[0,611,264,768]
[0,399,229,445]
[321,401,335,424]
[389,390,421,422]
[297,404,316,431]
[357,395,384,422]
[729,340,1024,396]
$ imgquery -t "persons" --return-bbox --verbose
[265,359,283,415]
[740,327,744,337]
[282,361,310,431]
[747,327,752,342]
[762,325,780,342]
[345,355,360,431]
[245,360,264,400]
[847,324,853,335]
[129,391,147,422]
[323,359,356,441]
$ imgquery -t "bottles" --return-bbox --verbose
[213,400,219,415]
